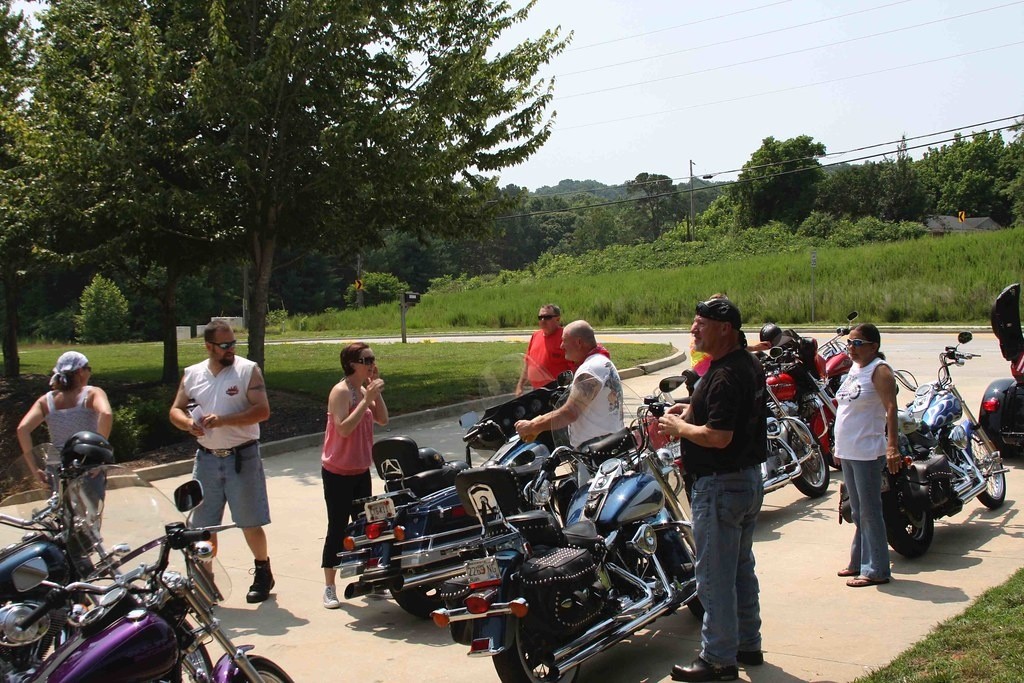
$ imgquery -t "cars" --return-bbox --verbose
[977,283,1024,462]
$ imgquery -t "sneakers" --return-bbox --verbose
[323,586,340,609]
[365,589,393,599]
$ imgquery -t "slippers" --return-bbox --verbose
[847,575,890,587]
[837,568,860,576]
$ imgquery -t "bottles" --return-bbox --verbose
[186,397,208,441]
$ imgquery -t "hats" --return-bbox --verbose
[695,298,741,324]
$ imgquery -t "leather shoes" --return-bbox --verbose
[670,656,738,681]
[736,651,763,665]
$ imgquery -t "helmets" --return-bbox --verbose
[759,323,783,347]
[61,432,119,478]
[418,447,445,472]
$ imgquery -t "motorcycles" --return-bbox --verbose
[0,438,126,683]
[631,307,862,508]
[0,465,299,683]
[430,372,705,683]
[330,352,628,621]
[838,331,1010,560]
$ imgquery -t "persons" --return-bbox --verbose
[321,342,394,608]
[516,305,610,398]
[170,319,275,603]
[658,299,768,683]
[834,324,902,587]
[690,294,771,377]
[514,320,624,487]
[18,351,113,523]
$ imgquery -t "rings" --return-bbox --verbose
[210,422,212,425]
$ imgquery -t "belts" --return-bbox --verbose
[196,440,260,474]
[691,463,759,480]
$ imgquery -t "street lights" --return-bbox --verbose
[689,158,697,244]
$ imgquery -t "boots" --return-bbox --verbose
[245,556,275,603]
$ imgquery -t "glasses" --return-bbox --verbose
[81,367,92,372]
[845,338,874,347]
[537,315,558,320]
[351,356,377,366]
[208,340,236,349]
